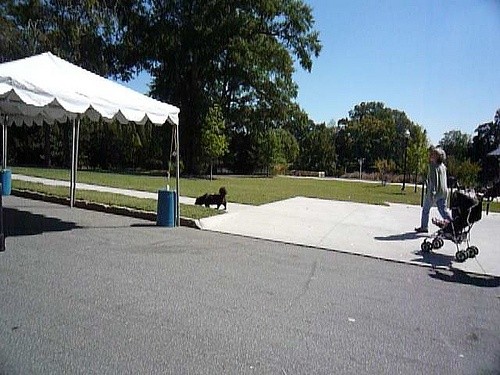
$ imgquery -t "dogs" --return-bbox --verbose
[195,187,228,211]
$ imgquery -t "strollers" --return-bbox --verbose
[421,190,484,263]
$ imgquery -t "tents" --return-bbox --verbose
[0,50,180,229]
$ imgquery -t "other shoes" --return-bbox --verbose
[414,227,428,233]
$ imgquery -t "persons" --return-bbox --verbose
[414,149,453,233]
[431,217,450,228]
